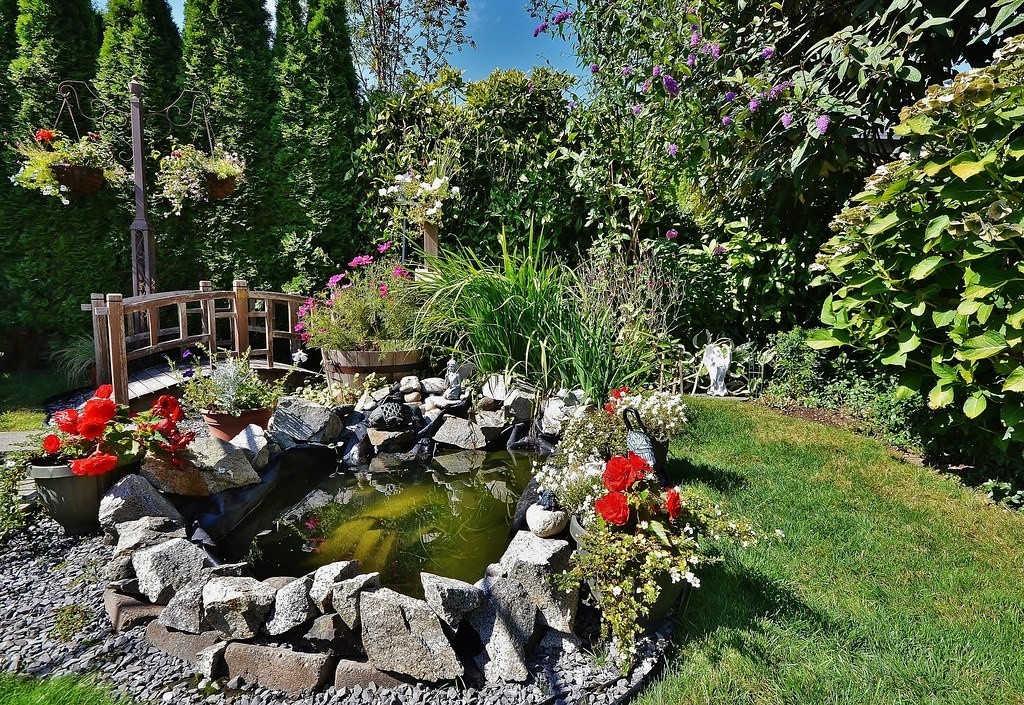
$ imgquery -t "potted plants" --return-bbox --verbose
[48,328,97,388]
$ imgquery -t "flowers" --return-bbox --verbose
[547,379,692,456]
[1,117,134,206]
[533,457,786,674]
[292,241,435,350]
[160,346,308,411]
[148,132,250,219]
[32,384,198,478]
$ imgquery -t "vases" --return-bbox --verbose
[648,433,670,462]
[570,510,685,625]
[199,408,273,442]
[32,447,147,536]
[41,161,104,196]
[190,172,237,199]
[320,344,426,404]
